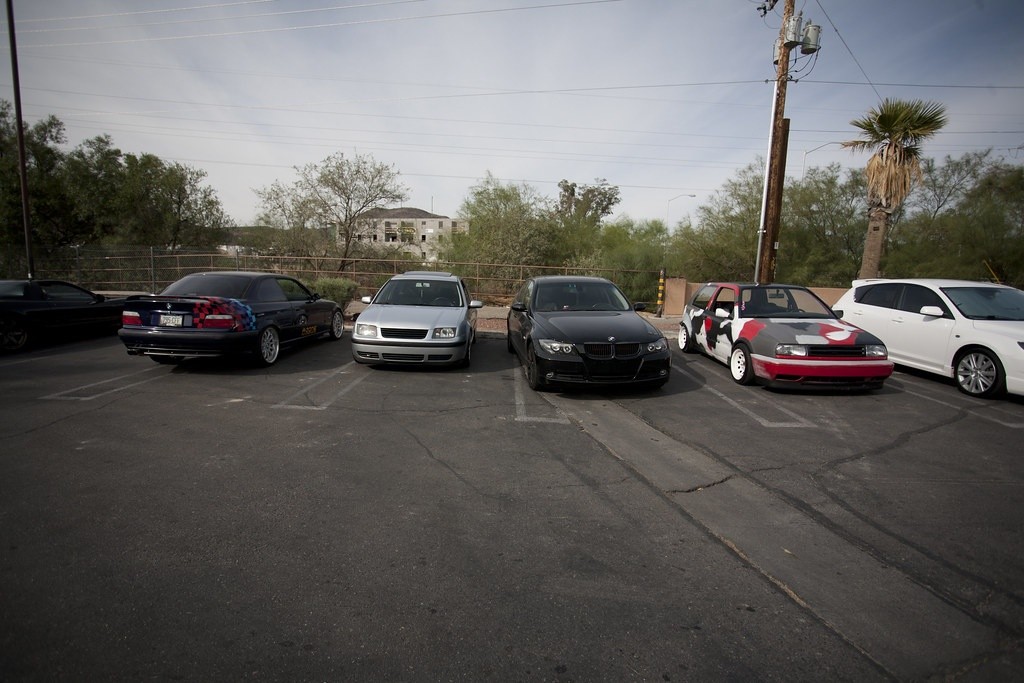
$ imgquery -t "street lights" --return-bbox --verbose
[664,193,697,229]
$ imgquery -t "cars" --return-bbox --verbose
[0,280,129,354]
[117,270,345,370]
[830,278,1024,397]
[677,282,895,392]
[633,302,657,312]
[507,275,672,391]
[351,270,483,367]
